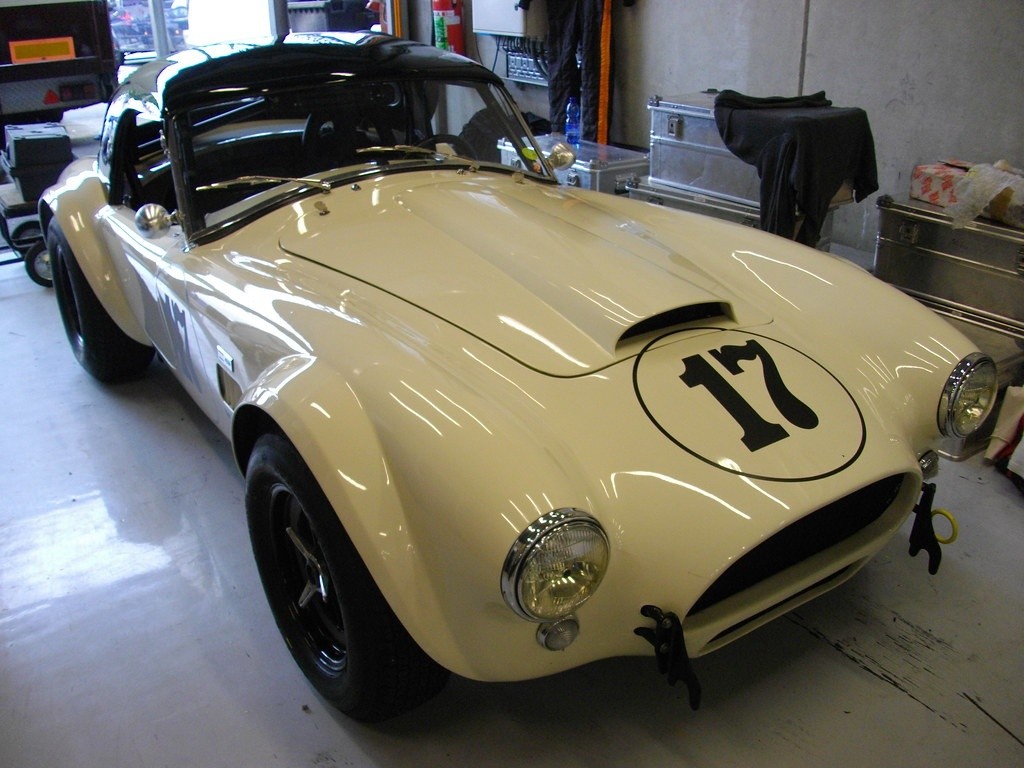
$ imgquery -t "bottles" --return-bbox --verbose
[564,97,580,144]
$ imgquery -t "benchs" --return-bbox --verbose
[144,131,375,212]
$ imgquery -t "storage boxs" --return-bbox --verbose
[0,123,76,204]
[496,89,853,253]
[873,161,1024,460]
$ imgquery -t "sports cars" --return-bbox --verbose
[39,37,1002,733]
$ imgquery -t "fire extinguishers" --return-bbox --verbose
[432,0,464,57]
